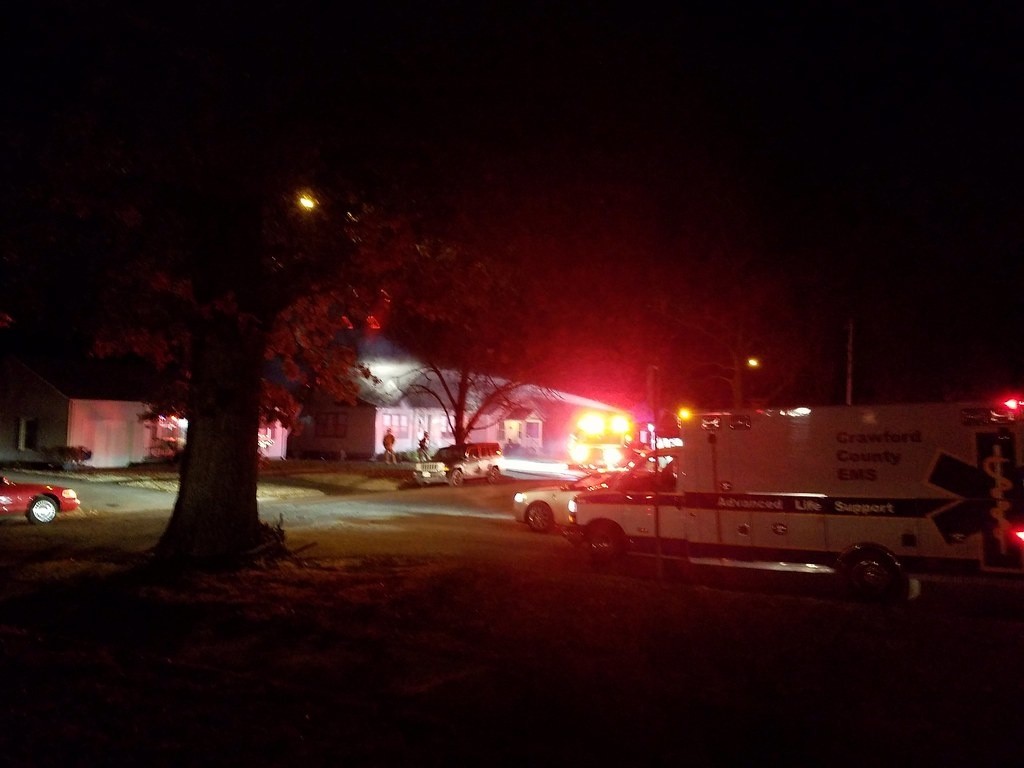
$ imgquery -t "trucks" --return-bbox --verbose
[562,398,1024,604]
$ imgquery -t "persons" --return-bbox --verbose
[382,427,398,466]
[417,429,432,463]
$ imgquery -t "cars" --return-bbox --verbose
[0,474,81,526]
[513,470,618,534]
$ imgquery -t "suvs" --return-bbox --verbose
[413,442,505,489]
